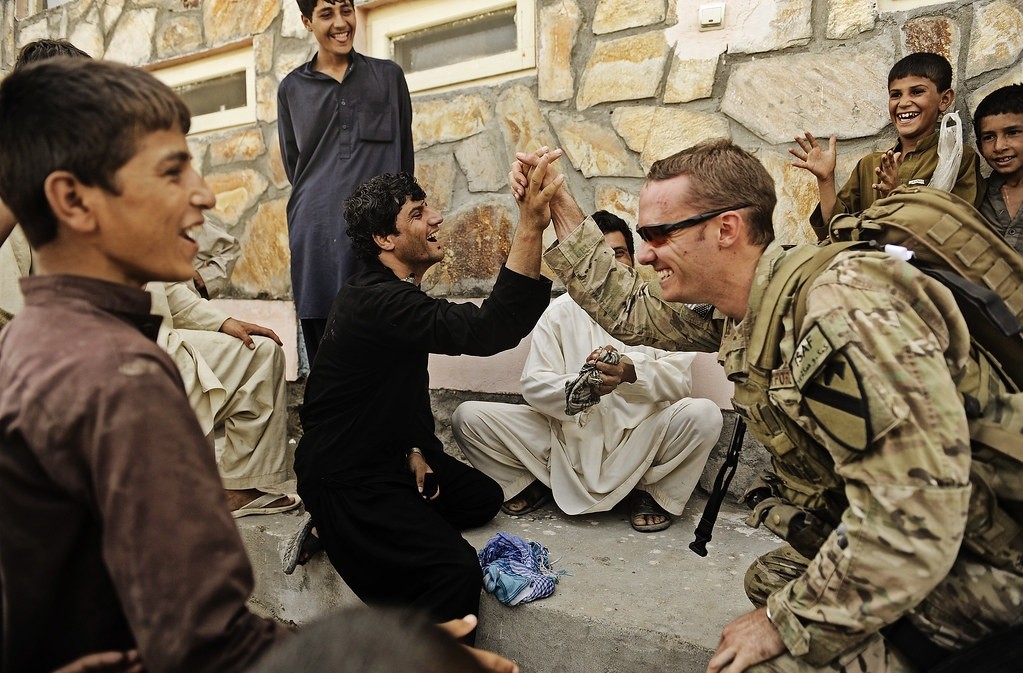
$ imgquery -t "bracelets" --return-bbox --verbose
[765,605,774,623]
[406,448,422,457]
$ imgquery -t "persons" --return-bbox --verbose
[508,136,1023,672]
[278,0,414,371]
[451,209,723,532]
[0,39,521,672]
[283,145,566,648]
[789,52,1023,264]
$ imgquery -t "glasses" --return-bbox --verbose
[635,202,754,248]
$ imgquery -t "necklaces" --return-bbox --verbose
[1004,188,1011,206]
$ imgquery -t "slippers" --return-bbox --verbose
[283,511,322,575]
[500,480,552,516]
[630,490,672,531]
[224,486,302,519]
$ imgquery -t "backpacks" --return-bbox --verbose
[793,185,1023,464]
